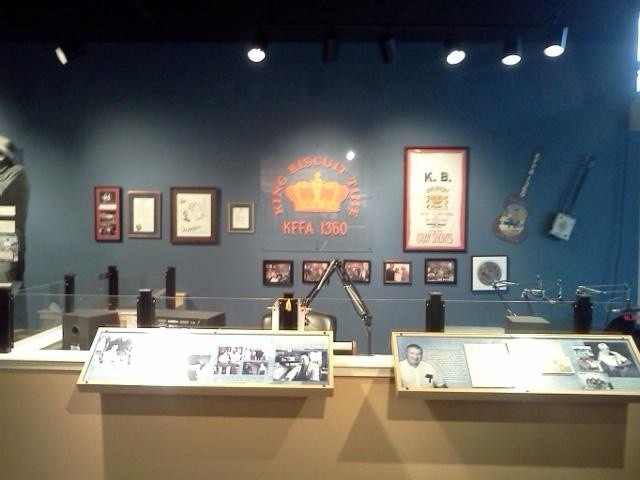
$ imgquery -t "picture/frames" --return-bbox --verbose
[76,327,335,399]
[403,149,469,252]
[262,260,292,286]
[302,260,328,283]
[224,203,256,233]
[168,187,218,244]
[125,187,162,238]
[262,131,376,252]
[384,261,412,285]
[423,258,455,285]
[95,185,122,242]
[341,259,372,284]
[389,332,640,399]
[472,254,510,294]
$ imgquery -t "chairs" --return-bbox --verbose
[264,309,337,342]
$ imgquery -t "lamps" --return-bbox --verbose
[502,37,521,66]
[56,42,81,66]
[378,34,396,61]
[443,35,466,66]
[244,27,277,63]
[492,278,562,334]
[574,280,639,334]
[269,257,373,355]
[544,20,568,57]
[322,30,337,60]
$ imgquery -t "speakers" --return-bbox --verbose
[62,309,120,351]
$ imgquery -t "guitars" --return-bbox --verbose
[492,148,543,245]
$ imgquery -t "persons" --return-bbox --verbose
[597,341,633,374]
[266,267,289,283]
[586,352,600,369]
[427,263,451,280]
[100,343,120,366]
[281,353,321,383]
[304,266,326,281]
[218,348,267,376]
[399,344,447,389]
[385,263,409,282]
[344,265,368,280]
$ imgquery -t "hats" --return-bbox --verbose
[598,343,610,352]
